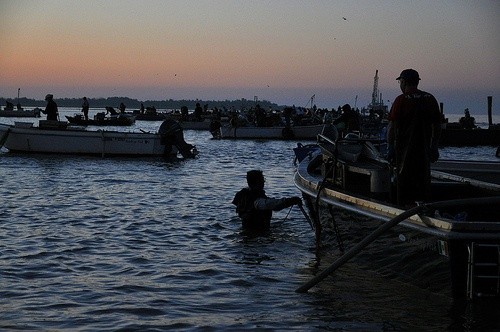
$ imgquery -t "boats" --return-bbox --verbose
[110,112,386,142]
[0,108,41,118]
[0,120,200,161]
[65,115,135,126]
[293,143,317,168]
[292,147,500,306]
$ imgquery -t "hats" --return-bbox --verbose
[396,69,421,82]
[247,170,265,183]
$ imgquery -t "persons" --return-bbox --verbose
[81,97,90,120]
[107,98,373,140]
[232,170,301,234]
[5,100,24,112]
[385,68,443,207]
[39,94,58,121]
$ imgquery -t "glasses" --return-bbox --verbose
[260,178,266,182]
[398,80,402,83]
